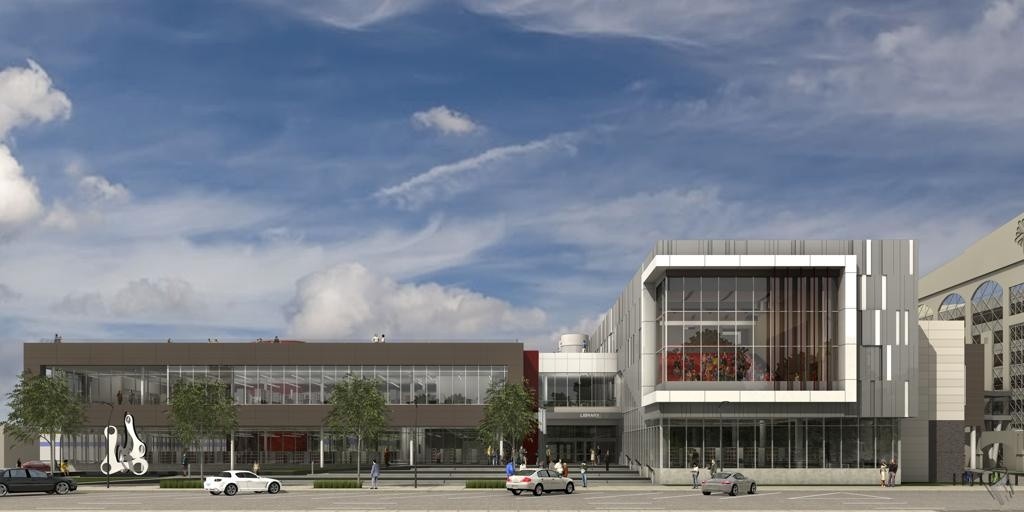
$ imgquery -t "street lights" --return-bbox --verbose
[405,393,418,486]
[90,400,113,487]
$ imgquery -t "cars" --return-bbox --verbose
[202,469,281,496]
[21,460,50,472]
[701,472,757,498]
[505,467,574,496]
[0,468,77,497]
